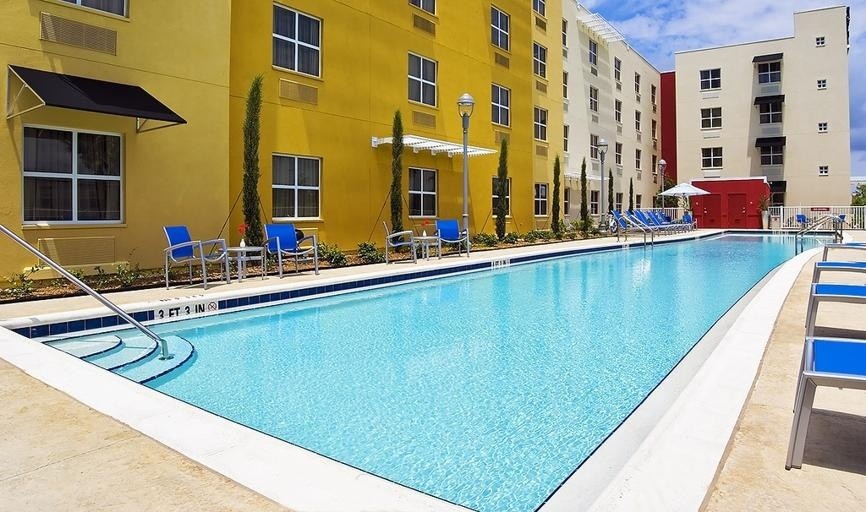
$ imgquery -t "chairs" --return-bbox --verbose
[161,220,324,287]
[610,207,697,242]
[784,211,866,477]
[382,217,472,263]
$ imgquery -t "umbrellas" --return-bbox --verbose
[657,182,711,212]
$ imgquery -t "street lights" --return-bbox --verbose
[456,92,476,230]
[658,158,667,210]
[597,139,608,220]
